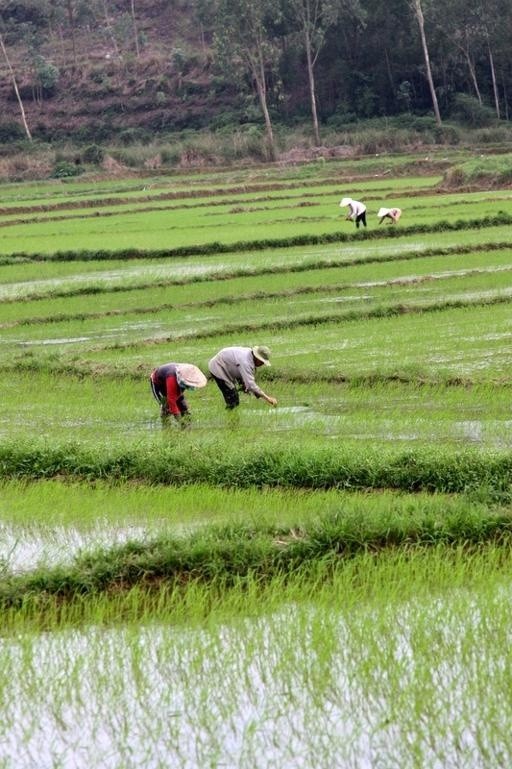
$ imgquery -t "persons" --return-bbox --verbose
[377,207,402,225]
[339,198,368,229]
[150,363,207,426]
[208,345,278,411]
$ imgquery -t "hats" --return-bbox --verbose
[252,345,272,367]
[176,363,208,388]
[339,198,352,208]
[378,208,389,217]
